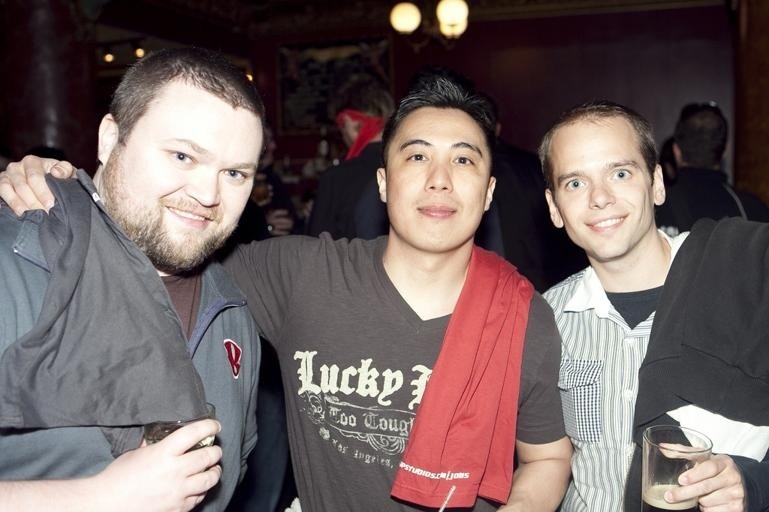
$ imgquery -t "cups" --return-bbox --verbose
[641,425,711,510]
[146,401,217,462]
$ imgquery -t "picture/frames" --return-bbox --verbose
[274,36,396,137]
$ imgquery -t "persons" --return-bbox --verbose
[0,79,576,512]
[654,101,768,237]
[521,97,769,510]
[216,122,301,511]
[306,75,510,260]
[0,36,262,510]
[467,89,555,297]
